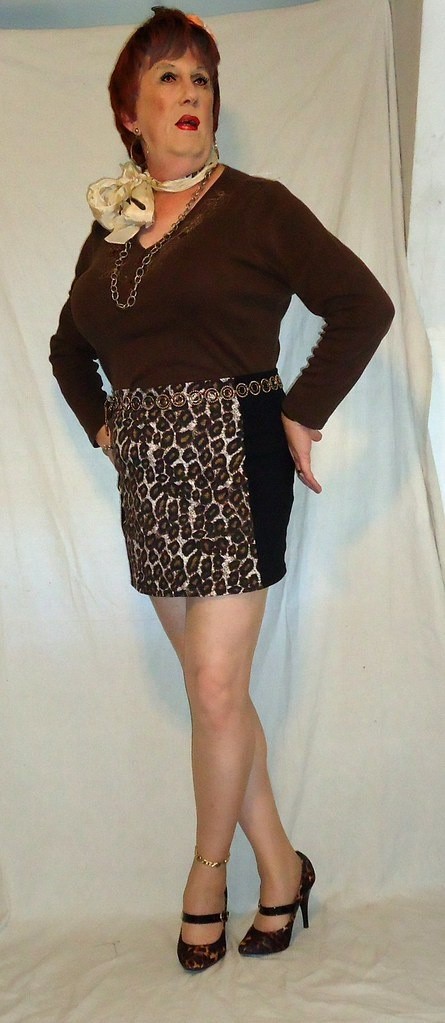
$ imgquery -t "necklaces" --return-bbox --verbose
[110,168,217,310]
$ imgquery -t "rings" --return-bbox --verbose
[298,471,303,476]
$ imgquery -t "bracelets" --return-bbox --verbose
[102,446,112,454]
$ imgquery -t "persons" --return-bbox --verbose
[49,5,396,974]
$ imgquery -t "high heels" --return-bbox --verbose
[176,885,229,974]
[237,850,316,957]
